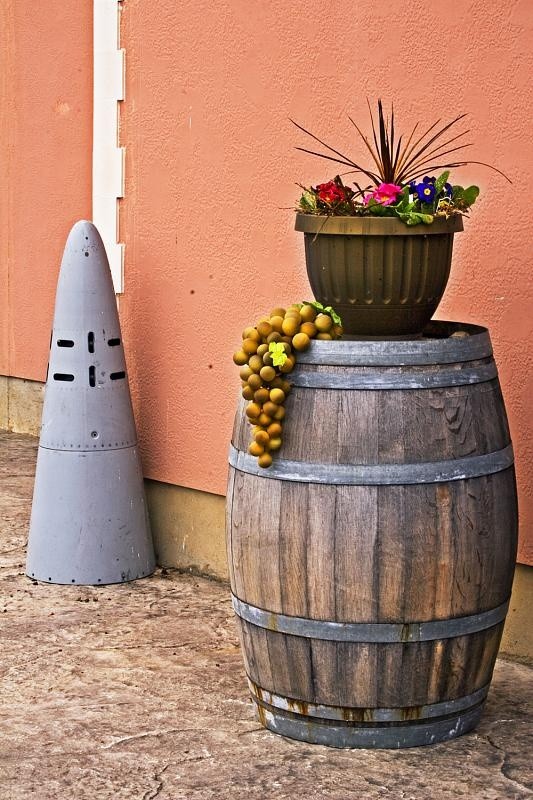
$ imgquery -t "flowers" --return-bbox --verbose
[287,96,512,228]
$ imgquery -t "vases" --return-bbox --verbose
[292,211,465,339]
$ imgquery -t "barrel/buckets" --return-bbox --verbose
[223,319,520,750]
